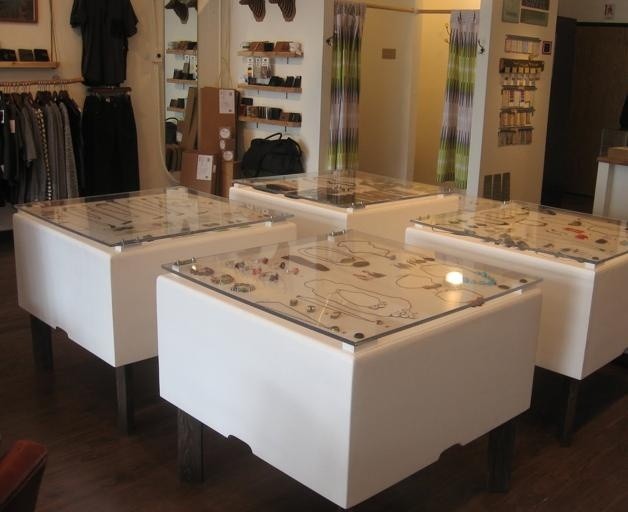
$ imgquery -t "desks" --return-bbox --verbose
[591,155,628,223]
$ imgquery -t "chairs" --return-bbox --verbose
[0,438,50,511]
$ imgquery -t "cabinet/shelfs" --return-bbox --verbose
[166,46,197,112]
[237,51,302,128]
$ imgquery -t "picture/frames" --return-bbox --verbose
[0,0,39,23]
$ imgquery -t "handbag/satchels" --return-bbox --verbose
[240,132,303,179]
[166,117,178,144]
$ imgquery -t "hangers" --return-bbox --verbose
[0,79,70,103]
